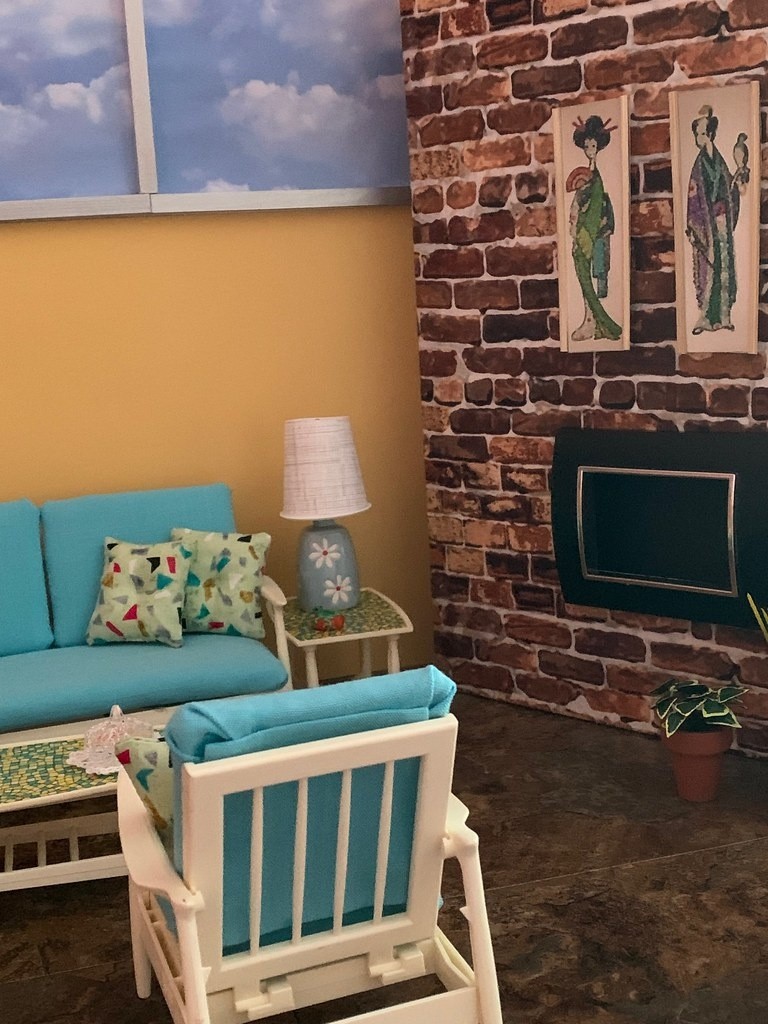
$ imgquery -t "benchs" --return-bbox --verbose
[0,483,293,745]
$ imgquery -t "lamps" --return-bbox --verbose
[280,416,372,610]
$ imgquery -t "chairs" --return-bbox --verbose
[111,665,504,1024]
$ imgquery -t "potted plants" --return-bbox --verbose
[647,678,751,802]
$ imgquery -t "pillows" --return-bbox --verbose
[169,528,271,640]
[114,737,175,862]
[85,536,189,648]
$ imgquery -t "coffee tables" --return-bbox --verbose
[0,724,165,892]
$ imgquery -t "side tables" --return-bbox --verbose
[266,589,414,688]
